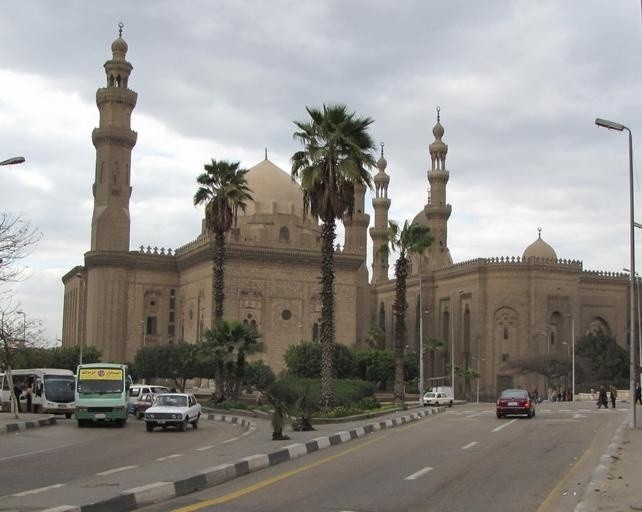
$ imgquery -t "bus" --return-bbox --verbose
[0,368,75,420]
[72,361,133,427]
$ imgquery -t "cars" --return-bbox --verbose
[144,393,202,431]
[495,389,536,419]
[423,392,455,408]
[127,392,156,418]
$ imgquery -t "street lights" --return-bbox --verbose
[472,356,486,404]
[16,310,27,350]
[0,156,25,166]
[450,288,465,403]
[140,320,147,346]
[563,341,570,360]
[621,267,641,398]
[594,117,638,428]
[566,314,575,402]
[419,309,429,403]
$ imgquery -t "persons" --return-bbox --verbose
[609,384,617,408]
[549,387,572,402]
[635,382,641,405]
[26,382,34,412]
[13,383,24,412]
[596,384,608,408]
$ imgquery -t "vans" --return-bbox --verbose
[126,384,171,416]
